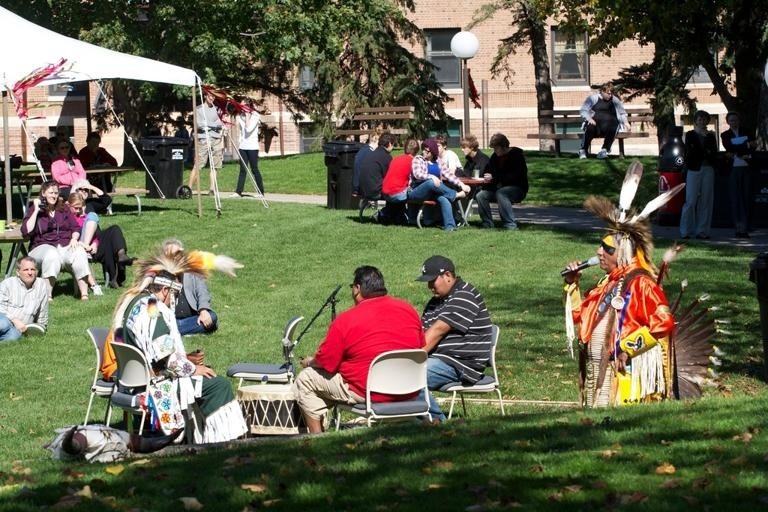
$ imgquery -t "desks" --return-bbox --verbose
[0,226,30,280]
[437,177,490,230]
[13,169,135,219]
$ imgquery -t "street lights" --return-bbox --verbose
[448,30,479,133]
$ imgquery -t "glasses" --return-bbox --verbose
[349,283,356,288]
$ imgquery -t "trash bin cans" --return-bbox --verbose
[323,142,365,209]
[143,137,192,199]
[750,253,767,381]
[656,138,686,226]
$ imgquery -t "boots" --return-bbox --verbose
[109,265,120,290]
[117,248,141,266]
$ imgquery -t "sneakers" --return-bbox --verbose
[578,149,588,160]
[595,149,609,159]
[89,282,105,297]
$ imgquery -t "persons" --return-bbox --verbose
[20,180,90,301]
[414,255,493,423]
[353,132,529,230]
[175,116,189,138]
[100,245,249,444]
[0,257,49,340]
[679,111,717,238]
[720,112,754,239]
[34,126,118,216]
[64,193,104,296]
[292,265,426,434]
[69,179,133,288]
[563,160,732,407]
[189,92,224,196]
[227,101,264,198]
[578,82,631,161]
[161,239,218,335]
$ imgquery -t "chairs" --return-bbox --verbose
[437,324,506,420]
[105,342,151,435]
[335,349,434,431]
[226,316,306,390]
[84,327,117,425]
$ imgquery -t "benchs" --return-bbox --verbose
[526,109,655,160]
[351,193,437,229]
[28,188,151,216]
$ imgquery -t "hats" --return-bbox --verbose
[487,133,509,149]
[414,254,458,285]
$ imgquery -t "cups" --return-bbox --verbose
[0,220,5,234]
[473,169,480,178]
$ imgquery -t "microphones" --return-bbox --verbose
[282,338,290,371]
[561,256,600,276]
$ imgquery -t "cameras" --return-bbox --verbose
[38,195,49,208]
